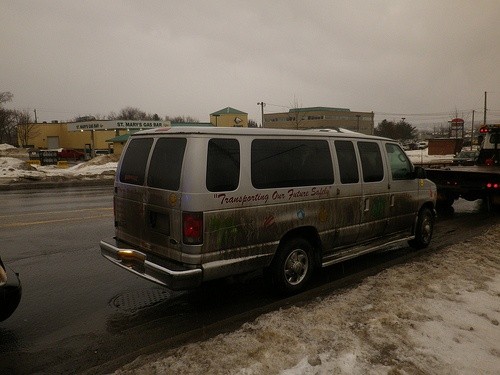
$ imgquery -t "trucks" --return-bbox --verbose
[419,124,500,218]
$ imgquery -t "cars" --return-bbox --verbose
[450,149,479,165]
[401,141,428,150]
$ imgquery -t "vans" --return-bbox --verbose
[0,257,22,322]
[99,122,438,297]
